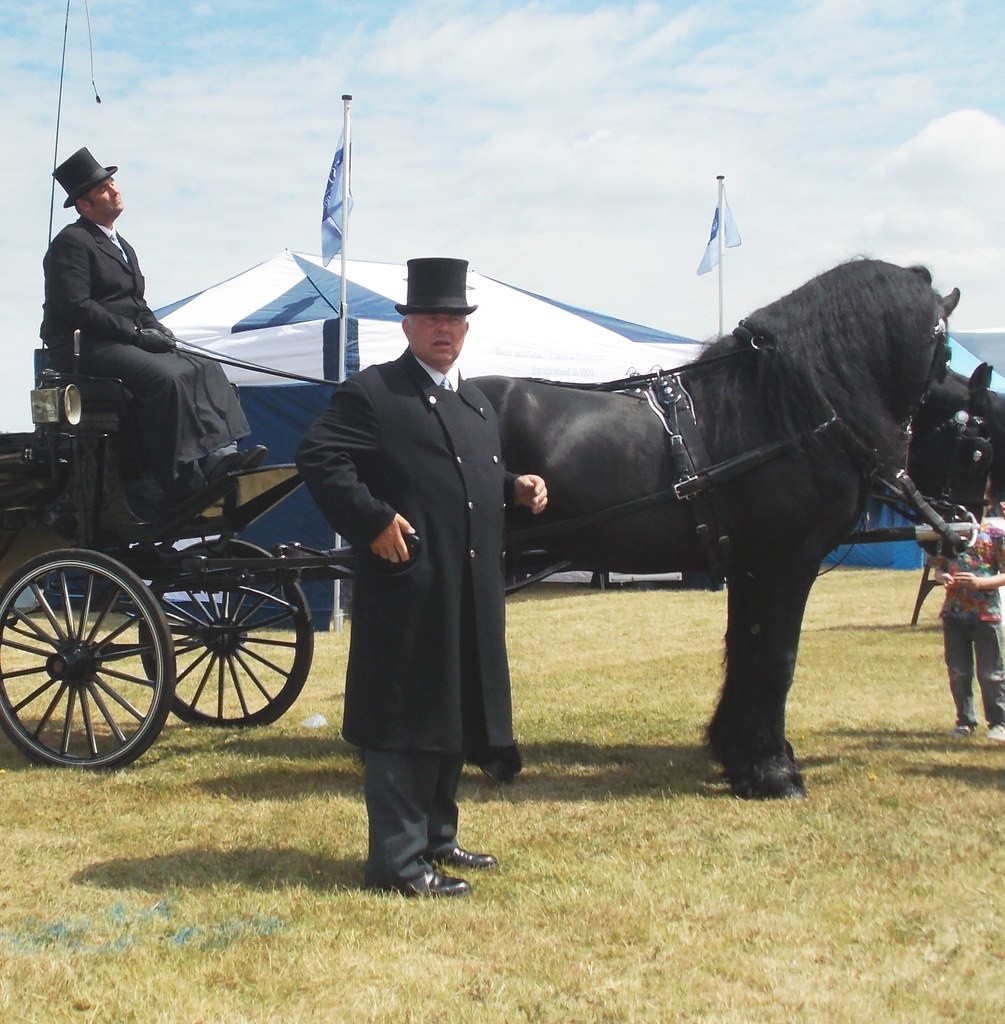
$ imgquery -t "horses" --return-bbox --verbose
[465,253,1004,802]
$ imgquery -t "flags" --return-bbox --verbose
[321,122,354,267]
[697,195,741,275]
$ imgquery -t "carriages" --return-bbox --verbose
[0,259,1005,801]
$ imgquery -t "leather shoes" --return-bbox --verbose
[432,847,496,868]
[375,871,470,898]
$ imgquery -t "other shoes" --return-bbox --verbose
[238,445,268,469]
[954,725,972,737]
[207,452,241,482]
[989,724,1005,740]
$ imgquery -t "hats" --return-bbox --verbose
[52,147,118,208]
[395,257,478,315]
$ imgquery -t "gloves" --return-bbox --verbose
[146,322,174,339]
[131,329,174,353]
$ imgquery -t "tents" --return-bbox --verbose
[47,251,1005,631]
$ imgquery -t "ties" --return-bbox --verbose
[110,235,117,242]
[440,377,452,390]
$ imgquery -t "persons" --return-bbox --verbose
[296,258,548,897]
[41,146,267,511]
[935,481,1005,740]
